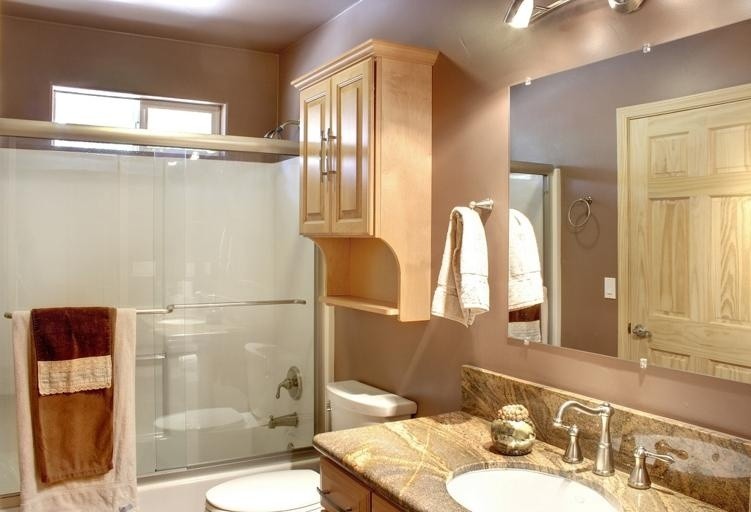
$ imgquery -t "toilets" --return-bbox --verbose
[155,343,282,472]
[205,379,417,512]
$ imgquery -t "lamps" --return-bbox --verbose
[502,0,643,28]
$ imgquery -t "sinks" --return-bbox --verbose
[610,435,751,477]
[445,462,623,512]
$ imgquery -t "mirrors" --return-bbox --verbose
[509,17,751,384]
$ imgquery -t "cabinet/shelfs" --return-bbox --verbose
[290,39,439,322]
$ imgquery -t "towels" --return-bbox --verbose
[32,307,112,396]
[11,306,137,512]
[29,307,117,484]
[508,304,549,342]
[509,208,544,311]
[431,206,490,328]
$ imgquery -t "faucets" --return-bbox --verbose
[552,400,616,476]
